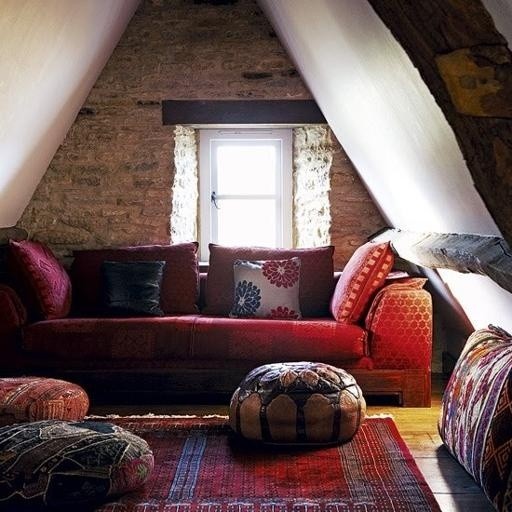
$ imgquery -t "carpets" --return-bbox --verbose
[84,412,443,512]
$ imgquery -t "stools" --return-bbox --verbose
[229,361,366,446]
[1,378,154,511]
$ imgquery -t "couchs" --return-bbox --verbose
[0,270,435,409]
